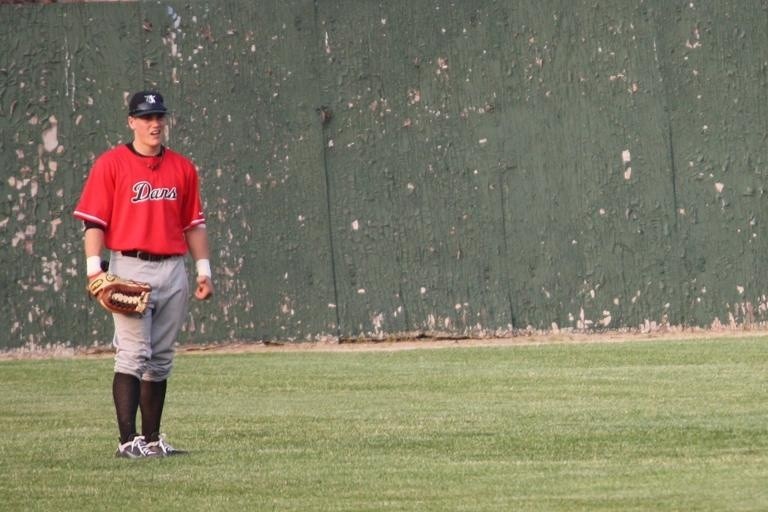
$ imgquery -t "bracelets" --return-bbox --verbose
[86,255,101,276]
[195,259,211,279]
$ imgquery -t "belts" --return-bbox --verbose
[120,249,182,263]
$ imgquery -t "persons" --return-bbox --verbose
[73,91,214,458]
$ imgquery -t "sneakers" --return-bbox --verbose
[147,436,188,456]
[114,436,162,458]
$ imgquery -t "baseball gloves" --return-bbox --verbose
[86,271,152,319]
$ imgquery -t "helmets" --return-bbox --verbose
[129,90,168,117]
